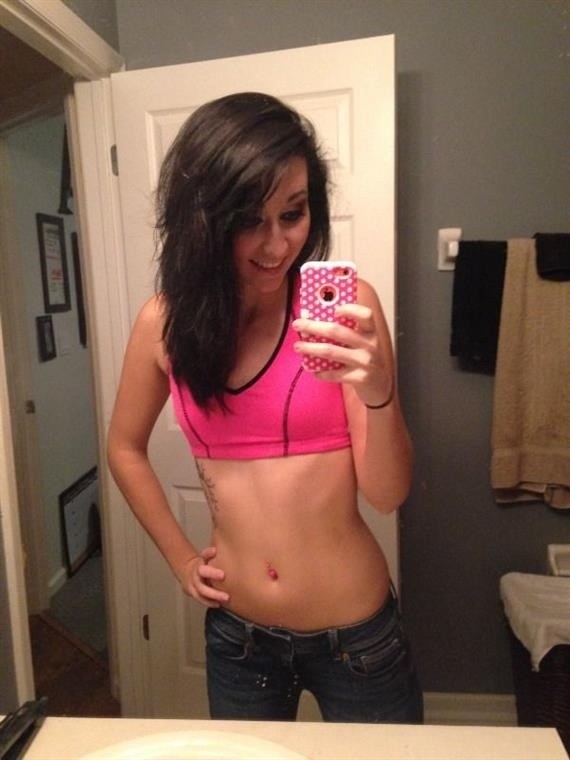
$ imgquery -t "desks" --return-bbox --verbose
[500,571,569,715]
[1,712,569,759]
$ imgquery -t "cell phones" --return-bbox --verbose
[300,260,358,373]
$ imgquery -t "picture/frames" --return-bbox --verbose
[36,212,72,312]
[58,466,101,578]
[36,315,56,362]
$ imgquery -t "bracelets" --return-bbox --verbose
[364,374,395,409]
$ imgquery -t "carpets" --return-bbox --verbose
[296,694,520,726]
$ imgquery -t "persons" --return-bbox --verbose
[106,93,424,725]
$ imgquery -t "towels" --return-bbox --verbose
[446,231,570,514]
